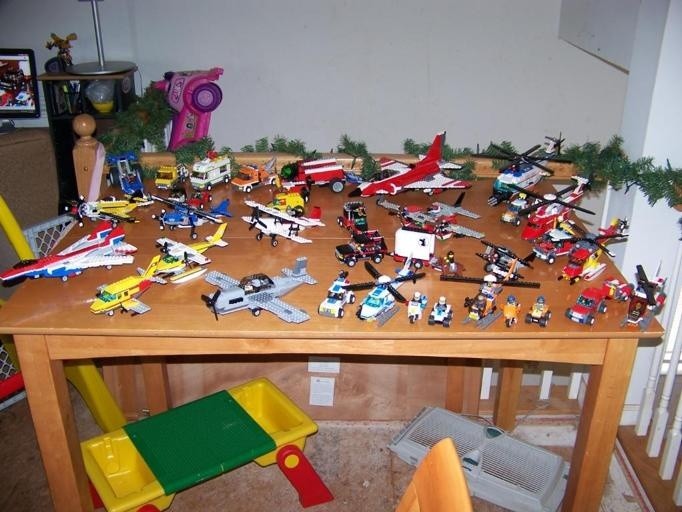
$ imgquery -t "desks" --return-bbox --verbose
[0,151,681,510]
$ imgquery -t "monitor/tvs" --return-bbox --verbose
[0,48,40,134]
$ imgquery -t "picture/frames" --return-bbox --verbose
[1,46,41,121]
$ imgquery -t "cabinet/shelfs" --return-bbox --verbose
[2,124,63,302]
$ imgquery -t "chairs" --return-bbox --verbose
[389,435,475,510]
